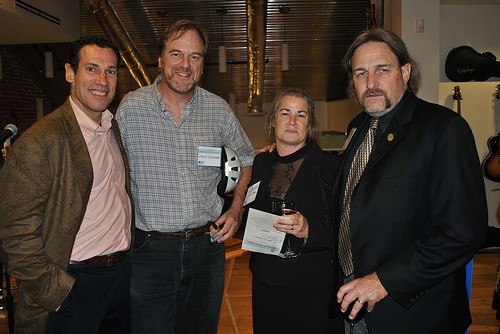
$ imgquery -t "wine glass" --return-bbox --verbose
[273,199,301,259]
[342,294,365,334]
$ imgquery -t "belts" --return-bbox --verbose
[68,251,126,266]
[136,225,214,240]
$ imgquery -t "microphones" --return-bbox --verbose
[0,124,18,146]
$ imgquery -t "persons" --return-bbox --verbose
[0,34,133,334]
[115,19,256,334]
[222,28,488,334]
[208,223,224,243]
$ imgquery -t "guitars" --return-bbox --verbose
[444,45,500,82]
[482,84,500,183]
[452,86,463,115]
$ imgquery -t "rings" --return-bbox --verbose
[357,299,364,305]
[291,225,293,230]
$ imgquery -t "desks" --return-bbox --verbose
[217,237,250,334]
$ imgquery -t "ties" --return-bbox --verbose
[338,117,380,277]
